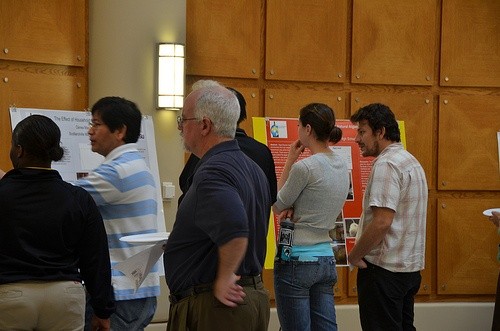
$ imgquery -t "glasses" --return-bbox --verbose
[89,121,105,129]
[177,115,212,124]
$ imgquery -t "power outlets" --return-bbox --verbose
[157,181,178,206]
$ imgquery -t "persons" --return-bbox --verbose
[488,212,500,331]
[272,102,350,331]
[348,103,428,331]
[68,96,161,331]
[0,115,117,331]
[162,79,278,331]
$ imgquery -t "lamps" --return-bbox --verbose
[156,43,186,111]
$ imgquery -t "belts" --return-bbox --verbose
[168,272,264,306]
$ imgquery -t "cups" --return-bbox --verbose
[279,221,295,261]
